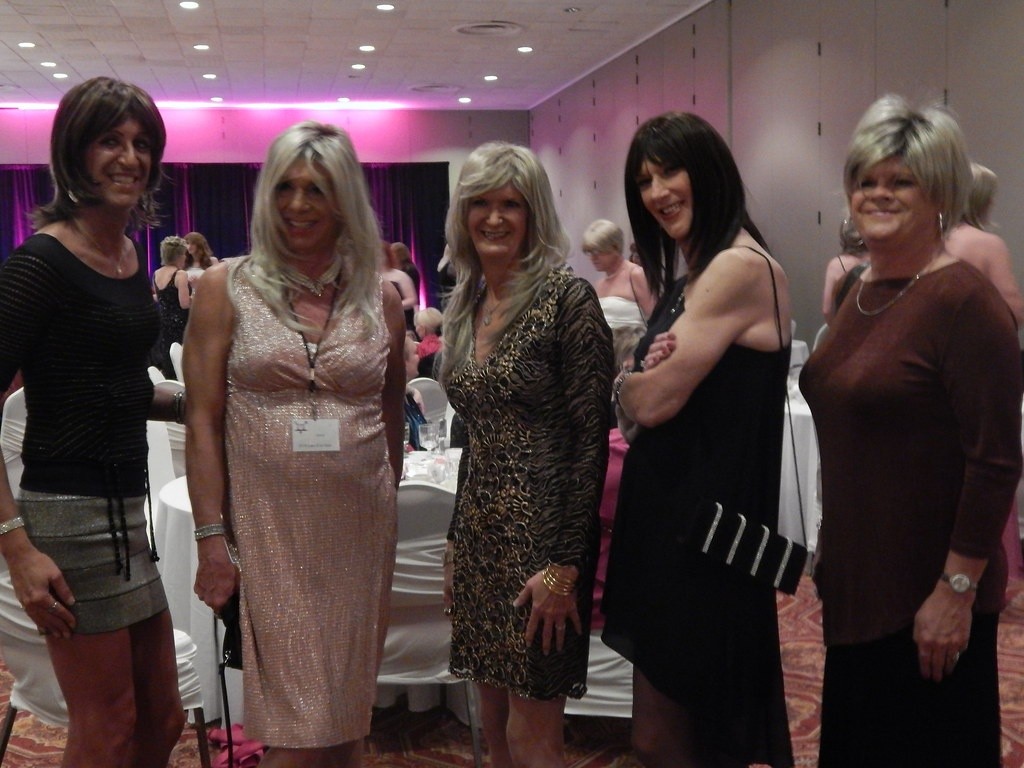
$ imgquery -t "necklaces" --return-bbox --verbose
[278,255,343,298]
[671,277,697,314]
[605,260,627,280]
[481,300,504,326]
[856,244,945,316]
[73,218,125,275]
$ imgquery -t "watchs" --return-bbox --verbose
[941,573,977,593]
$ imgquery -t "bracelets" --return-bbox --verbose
[542,564,575,596]
[441,551,454,567]
[173,390,186,425]
[194,524,225,541]
[0,517,25,535]
[616,371,632,402]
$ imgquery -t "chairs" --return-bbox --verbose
[0,339,213,768]
[407,378,447,450]
[376,476,634,768]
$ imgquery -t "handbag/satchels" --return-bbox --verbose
[218,593,242,672]
[671,498,809,607]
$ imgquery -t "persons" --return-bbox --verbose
[182,119,406,768]
[798,93,1024,768]
[0,76,186,768]
[600,111,796,768]
[149,232,220,380]
[581,219,658,389]
[380,240,457,454]
[436,142,615,768]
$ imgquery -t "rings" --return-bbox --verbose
[555,624,566,630]
[443,608,451,616]
[950,652,960,663]
[48,601,59,612]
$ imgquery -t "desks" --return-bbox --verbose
[779,383,821,555]
[155,449,468,728]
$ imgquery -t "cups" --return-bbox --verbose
[404,421,410,444]
[445,447,463,481]
[425,463,444,483]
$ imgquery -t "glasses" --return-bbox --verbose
[584,249,601,256]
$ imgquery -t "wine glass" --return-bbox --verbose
[419,423,439,461]
